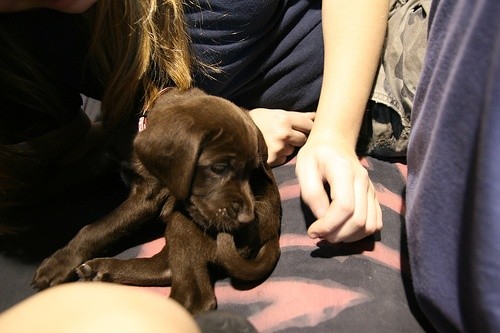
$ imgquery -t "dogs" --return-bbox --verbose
[30,87,282,317]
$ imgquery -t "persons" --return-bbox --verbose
[399,0,500,332]
[108,0,392,246]
[1,0,162,269]
